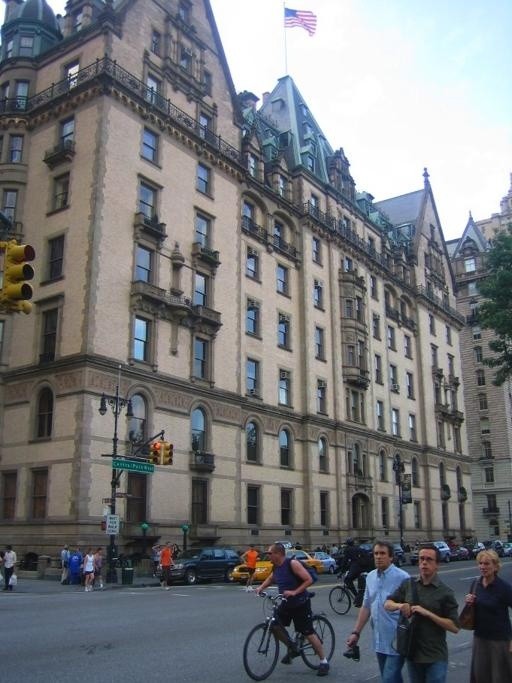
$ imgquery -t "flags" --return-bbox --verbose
[285,8,316,36]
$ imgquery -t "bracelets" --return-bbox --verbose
[352,632,359,637]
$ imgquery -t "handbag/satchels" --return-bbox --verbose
[63,559,69,568]
[8,571,18,586]
[397,621,415,659]
[457,602,478,630]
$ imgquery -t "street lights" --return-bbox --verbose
[97,360,136,577]
[391,453,408,549]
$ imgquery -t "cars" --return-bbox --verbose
[307,550,336,574]
[155,545,243,583]
[231,539,323,585]
[410,539,511,562]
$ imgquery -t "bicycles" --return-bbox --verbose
[241,588,337,680]
[329,573,369,616]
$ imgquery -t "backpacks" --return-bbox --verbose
[355,546,373,570]
[297,560,318,588]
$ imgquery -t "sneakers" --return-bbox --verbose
[352,594,364,607]
[316,661,330,677]
[85,582,105,592]
[162,586,170,591]
[281,650,299,665]
[245,585,255,594]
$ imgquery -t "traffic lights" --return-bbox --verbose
[162,441,173,464]
[148,444,154,464]
[154,440,164,463]
[0,241,37,307]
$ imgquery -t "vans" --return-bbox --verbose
[332,541,405,570]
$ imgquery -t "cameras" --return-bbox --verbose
[343,645,359,660]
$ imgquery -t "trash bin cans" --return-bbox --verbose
[123,568,134,584]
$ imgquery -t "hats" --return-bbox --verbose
[343,537,357,546]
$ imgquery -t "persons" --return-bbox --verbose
[332,538,374,608]
[295,542,339,559]
[240,543,261,593]
[2,545,17,591]
[458,548,512,683]
[0,551,5,585]
[60,544,105,591]
[256,543,330,676]
[383,544,460,683]
[152,542,182,591]
[346,540,411,683]
